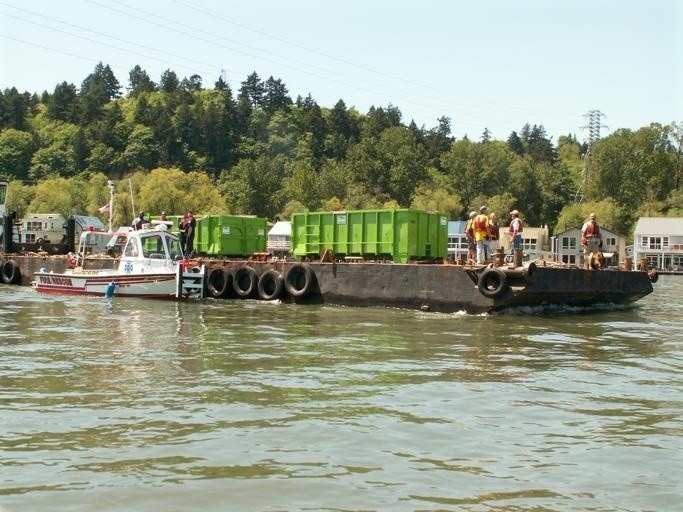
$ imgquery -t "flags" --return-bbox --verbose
[98,202,110,215]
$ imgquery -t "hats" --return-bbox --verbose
[479,206,487,210]
[510,210,519,215]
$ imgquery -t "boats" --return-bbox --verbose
[32,223,205,300]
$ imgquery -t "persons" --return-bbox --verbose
[132,212,147,230]
[155,210,169,256]
[473,205,492,264]
[464,211,478,264]
[183,211,196,254]
[580,213,603,269]
[508,210,523,268]
[178,214,187,254]
[487,212,500,260]
